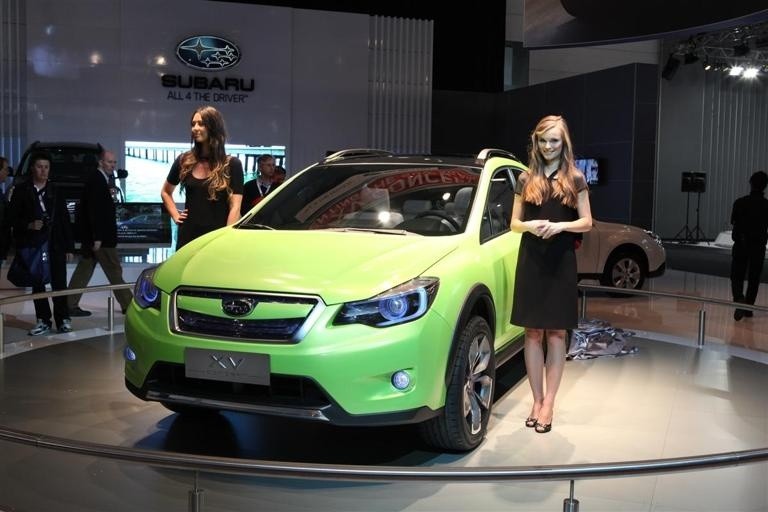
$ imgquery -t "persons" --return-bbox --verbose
[0,156,16,271]
[5,152,75,335]
[65,150,132,317]
[160,105,243,252]
[271,166,286,184]
[508,115,592,433]
[729,170,767,321]
[240,154,280,217]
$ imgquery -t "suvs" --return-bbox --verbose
[124,146,571,452]
[7,140,129,225]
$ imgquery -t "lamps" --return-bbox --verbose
[662,36,768,82]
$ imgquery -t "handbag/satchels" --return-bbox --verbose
[7,242,52,286]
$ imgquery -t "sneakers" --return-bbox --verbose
[29,318,52,336]
[59,319,73,332]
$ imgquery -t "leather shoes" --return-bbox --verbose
[67,308,91,317]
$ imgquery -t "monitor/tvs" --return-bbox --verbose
[573,155,607,188]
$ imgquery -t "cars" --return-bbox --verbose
[117,214,162,230]
[575,217,667,300]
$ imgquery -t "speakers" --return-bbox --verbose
[694,171,707,192]
[682,171,693,193]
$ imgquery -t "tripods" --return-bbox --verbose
[682,193,710,245]
[671,193,695,244]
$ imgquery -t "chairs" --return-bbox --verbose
[339,186,404,231]
[440,188,498,243]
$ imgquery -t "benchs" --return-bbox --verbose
[389,200,508,238]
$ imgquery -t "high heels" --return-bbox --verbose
[526,415,538,427]
[535,423,551,433]
